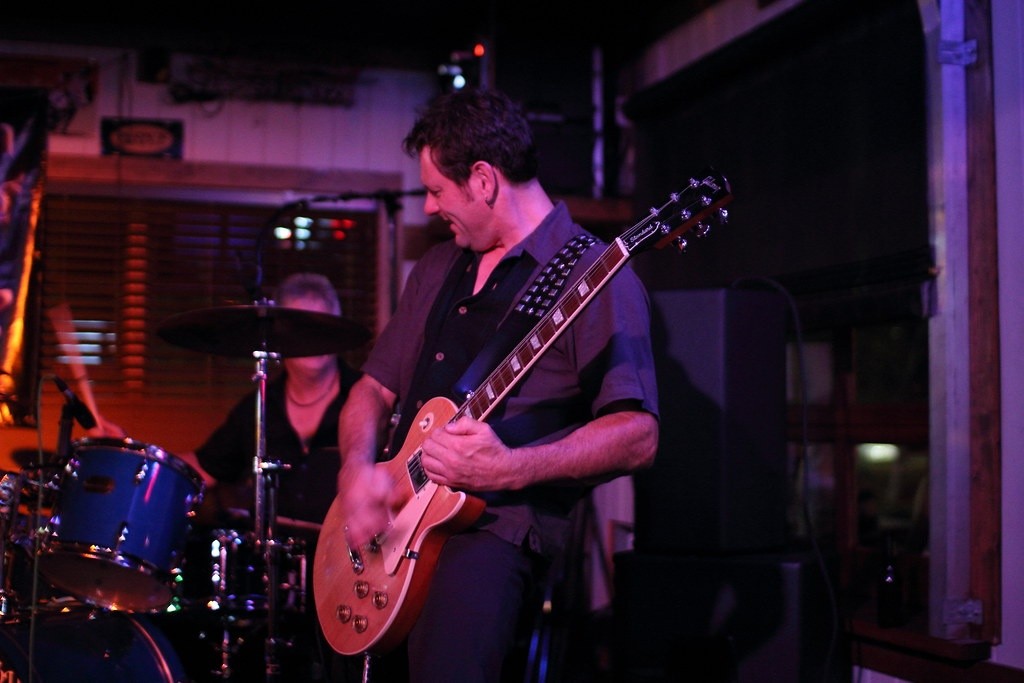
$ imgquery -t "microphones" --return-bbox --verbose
[51,375,97,430]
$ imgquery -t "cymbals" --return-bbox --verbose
[156,302,375,358]
[11,446,56,470]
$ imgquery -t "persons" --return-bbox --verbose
[337,92,660,683]
[74,275,363,683]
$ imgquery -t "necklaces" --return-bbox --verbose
[285,376,335,407]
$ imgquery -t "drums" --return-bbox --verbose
[2,595,189,682]
[34,435,208,613]
[0,471,55,567]
[185,529,308,619]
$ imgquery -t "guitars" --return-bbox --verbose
[310,171,739,660]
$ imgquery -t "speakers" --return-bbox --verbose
[616,286,788,553]
[606,549,854,683]
